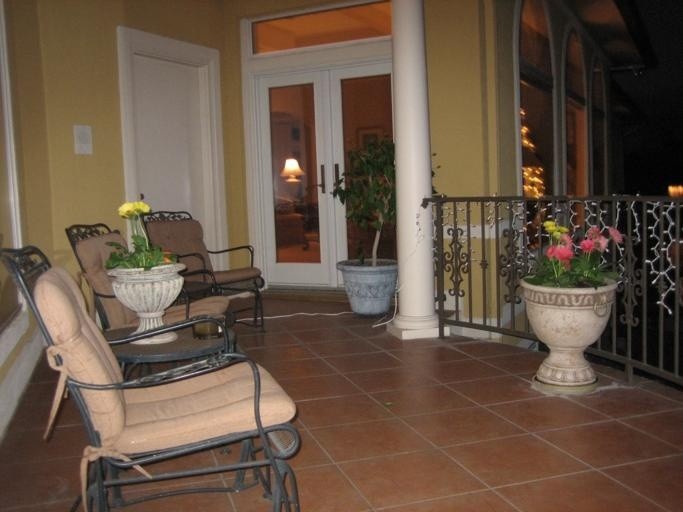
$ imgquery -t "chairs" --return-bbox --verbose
[0,244,301,512]
[138,210,265,335]
[275,196,309,251]
[64,223,219,375]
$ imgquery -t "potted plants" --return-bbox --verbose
[332,140,398,315]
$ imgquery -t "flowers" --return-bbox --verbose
[103,201,178,271]
[527,221,623,287]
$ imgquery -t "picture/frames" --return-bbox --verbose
[356,127,383,152]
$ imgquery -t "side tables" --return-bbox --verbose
[102,324,237,382]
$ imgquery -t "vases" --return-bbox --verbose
[106,262,188,345]
[518,274,618,395]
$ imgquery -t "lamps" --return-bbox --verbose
[280,157,304,183]
